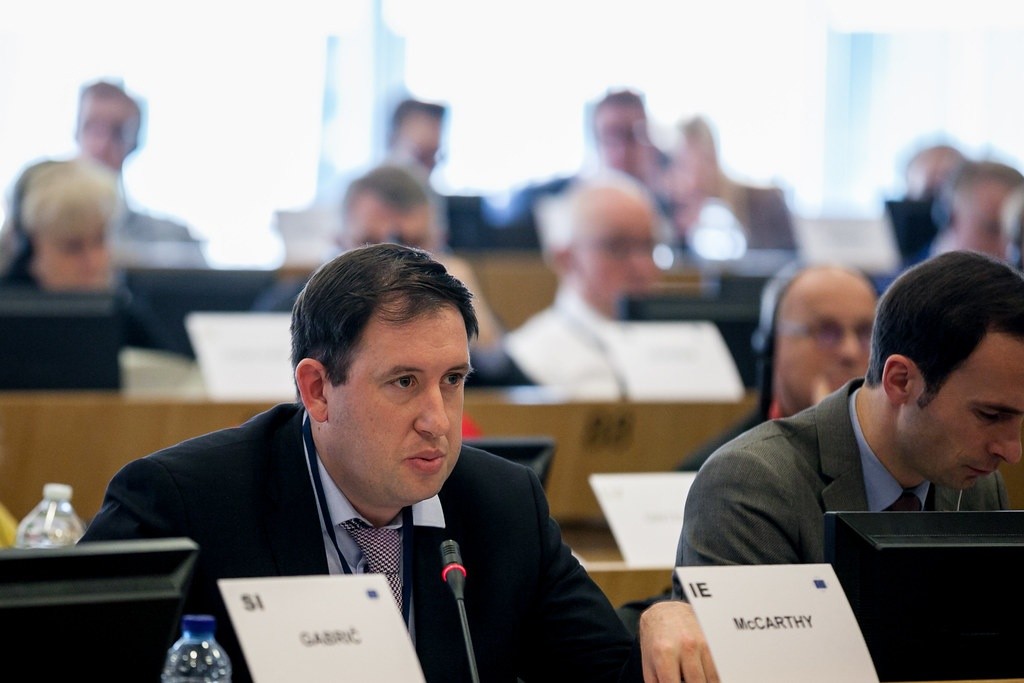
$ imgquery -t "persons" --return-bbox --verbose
[77,245,719,683]
[239,161,534,390]
[674,260,877,473]
[0,156,185,357]
[876,163,1024,293]
[382,98,495,249]
[1,79,209,268]
[900,147,971,218]
[657,114,798,249]
[494,170,746,400]
[619,248,1024,683]
[506,91,679,242]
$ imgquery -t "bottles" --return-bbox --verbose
[163,614,232,683]
[16,483,86,549]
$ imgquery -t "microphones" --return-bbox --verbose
[440,538,482,683]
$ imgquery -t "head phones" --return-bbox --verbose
[7,159,76,266]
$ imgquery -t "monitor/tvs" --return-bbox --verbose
[0,185,1024,683]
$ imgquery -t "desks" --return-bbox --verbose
[0,388,760,524]
[274,252,715,333]
